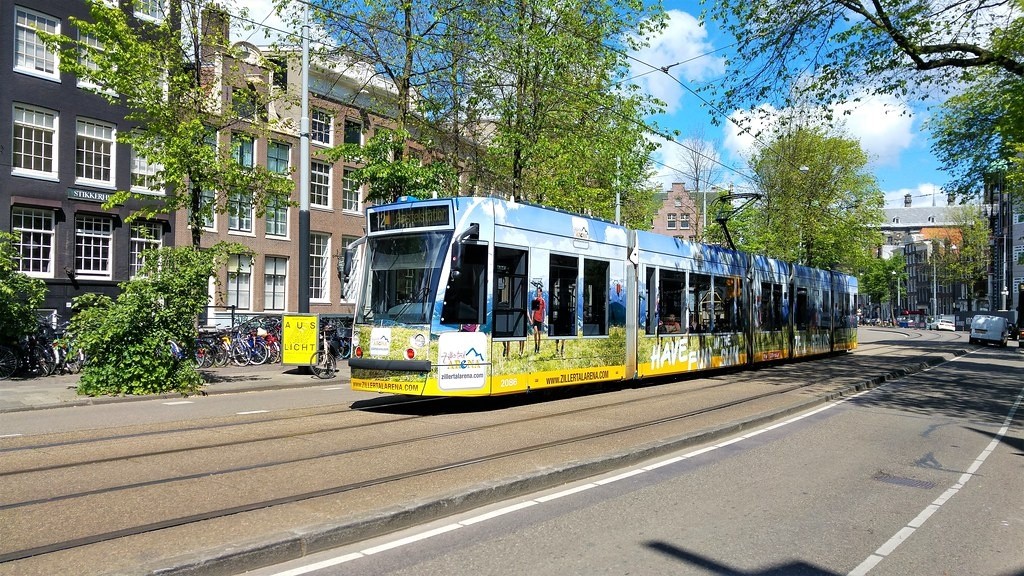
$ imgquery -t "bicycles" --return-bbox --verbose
[309,315,352,380]
[153,313,282,369]
[0,311,88,381]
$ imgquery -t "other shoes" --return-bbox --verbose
[502,346,511,360]
[555,349,564,357]
[534,344,540,353]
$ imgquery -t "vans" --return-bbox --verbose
[969,315,1012,348]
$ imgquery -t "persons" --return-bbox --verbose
[502,341,511,359]
[554,339,566,359]
[584,302,590,323]
[647,311,680,333]
[530,287,546,352]
[519,309,534,359]
[688,312,698,332]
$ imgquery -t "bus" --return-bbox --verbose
[342,192,858,398]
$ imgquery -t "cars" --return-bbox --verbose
[860,317,956,332]
